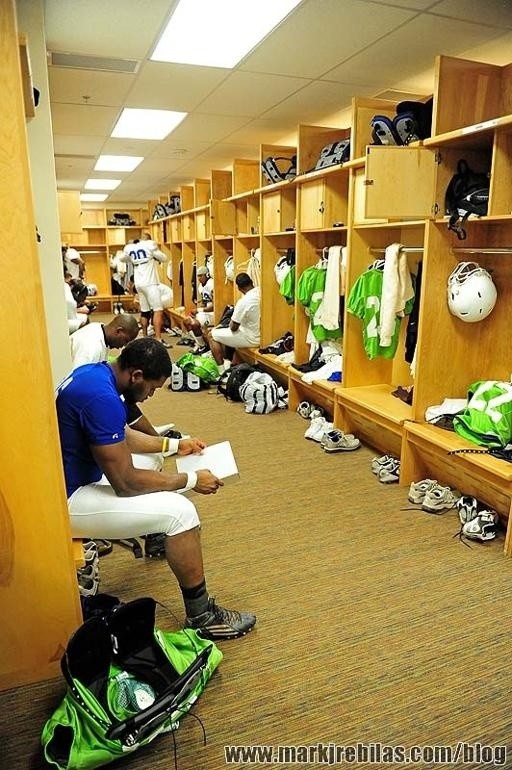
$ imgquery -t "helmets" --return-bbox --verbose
[224,256,234,282]
[274,256,292,285]
[447,261,498,323]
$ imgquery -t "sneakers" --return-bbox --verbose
[77,541,100,596]
[371,455,499,548]
[297,401,361,452]
[147,324,211,355]
[184,598,256,640]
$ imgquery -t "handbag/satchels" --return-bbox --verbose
[41,599,224,770]
[170,353,288,414]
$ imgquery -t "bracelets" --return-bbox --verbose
[169,439,179,452]
[161,437,168,454]
[186,471,197,488]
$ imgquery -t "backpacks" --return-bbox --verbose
[445,158,490,239]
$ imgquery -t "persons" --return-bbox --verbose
[54,337,259,643]
[207,271,261,385]
[179,266,215,355]
[62,233,176,350]
[69,313,183,441]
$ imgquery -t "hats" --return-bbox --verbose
[197,266,208,274]
